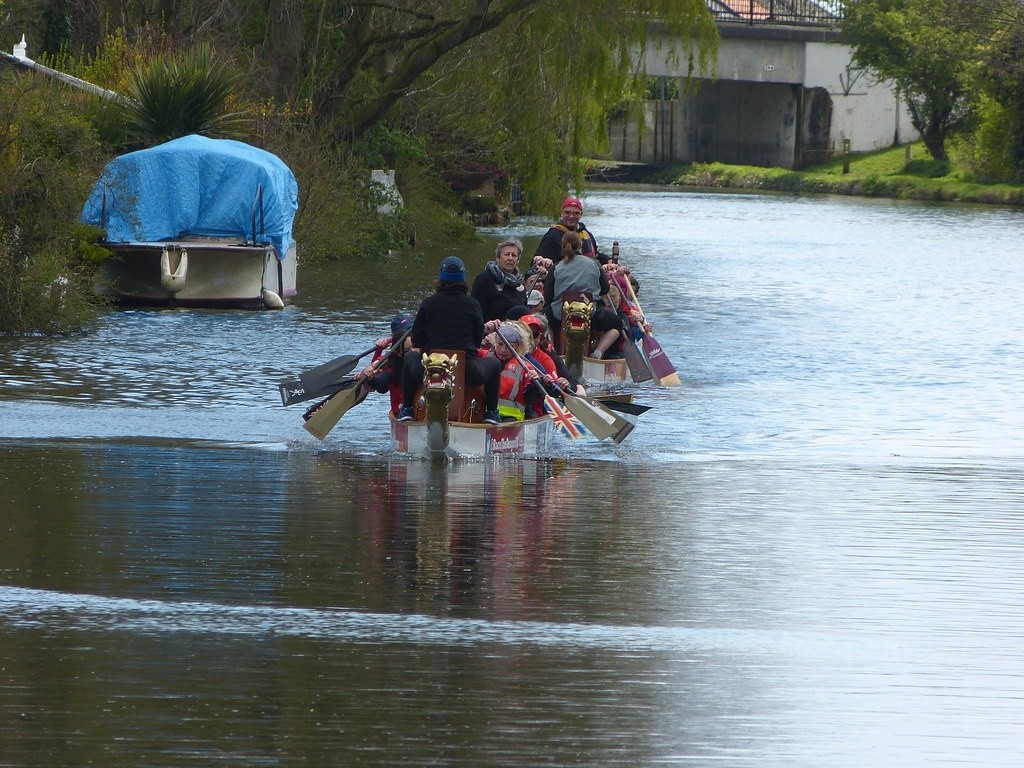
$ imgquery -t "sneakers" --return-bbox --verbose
[484,409,503,425]
[398,406,414,422]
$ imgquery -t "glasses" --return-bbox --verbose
[564,210,581,215]
[532,331,540,338]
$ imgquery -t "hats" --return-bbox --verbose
[524,269,537,282]
[562,198,583,210]
[439,256,465,272]
[520,314,542,333]
[391,315,414,332]
[526,290,545,305]
[505,306,532,321]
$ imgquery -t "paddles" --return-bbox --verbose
[278,267,680,439]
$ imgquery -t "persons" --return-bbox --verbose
[600,260,653,359]
[471,238,527,324]
[477,307,587,425]
[544,233,622,360]
[353,315,419,418]
[526,267,545,314]
[531,199,619,268]
[397,256,504,425]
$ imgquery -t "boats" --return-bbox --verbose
[385,348,555,461]
[93,135,298,312]
[550,292,632,384]
